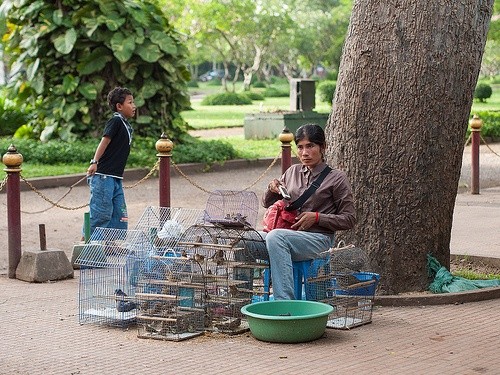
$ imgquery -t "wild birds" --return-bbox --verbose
[113,213,363,336]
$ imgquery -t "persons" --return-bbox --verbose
[81,88,136,254]
[224,123,357,315]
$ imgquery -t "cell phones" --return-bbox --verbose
[279,185,291,200]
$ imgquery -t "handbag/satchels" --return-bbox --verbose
[262,199,298,233]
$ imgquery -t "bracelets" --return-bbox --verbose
[90,159,100,165]
[314,210,318,224]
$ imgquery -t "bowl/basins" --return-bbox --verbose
[241,300,334,343]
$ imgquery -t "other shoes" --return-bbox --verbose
[105,248,135,256]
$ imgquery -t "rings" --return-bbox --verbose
[301,225,304,229]
